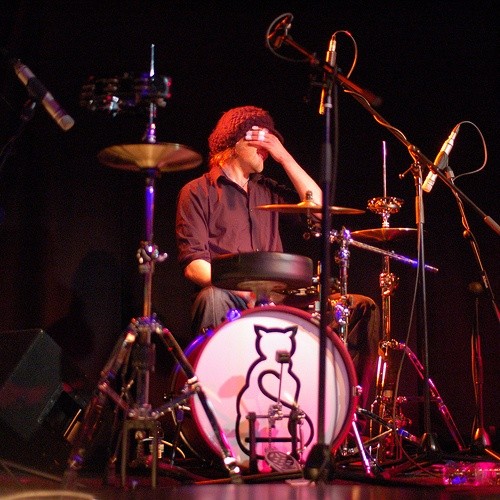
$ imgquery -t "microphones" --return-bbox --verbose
[266,15,292,41]
[420,125,460,193]
[318,36,337,115]
[13,60,75,131]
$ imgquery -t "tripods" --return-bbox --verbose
[356,213,470,461]
[68,164,241,486]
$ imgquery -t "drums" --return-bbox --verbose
[169,303,364,474]
[210,249,313,292]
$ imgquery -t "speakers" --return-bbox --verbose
[0,329,62,439]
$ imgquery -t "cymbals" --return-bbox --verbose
[251,201,366,214]
[350,227,417,244]
[80,74,171,111]
[96,142,202,172]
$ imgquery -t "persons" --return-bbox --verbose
[174,105,381,471]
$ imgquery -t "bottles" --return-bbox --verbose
[443,460,500,485]
[149,420,164,459]
